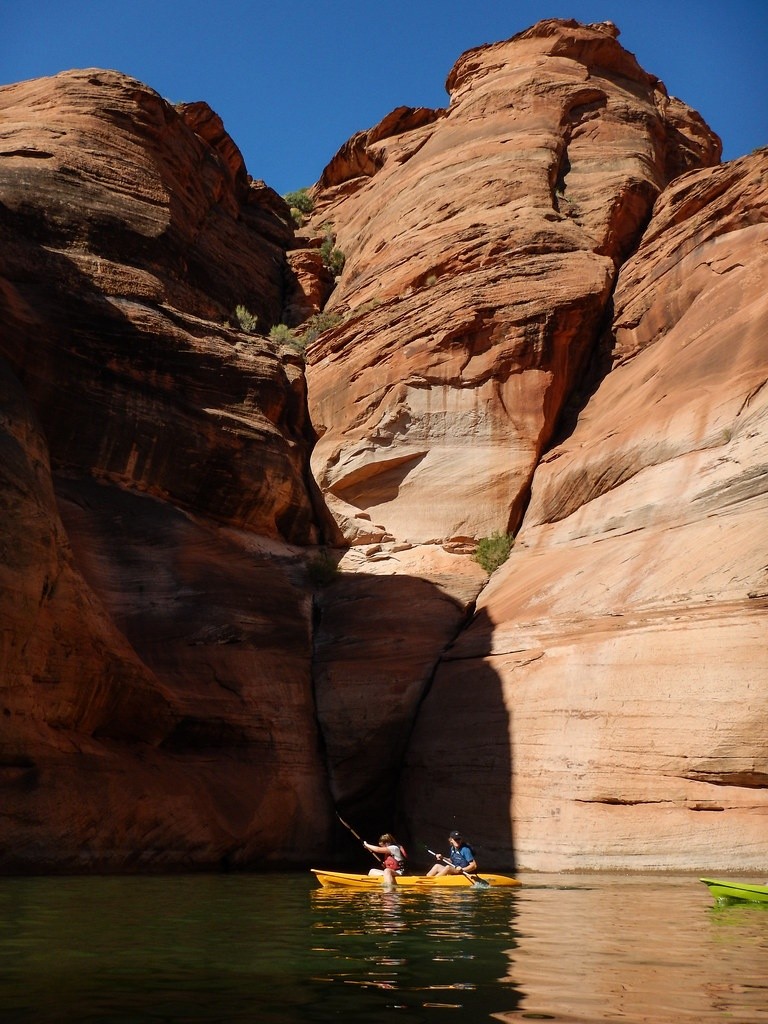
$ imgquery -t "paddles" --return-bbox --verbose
[334,810,383,864]
[414,837,490,886]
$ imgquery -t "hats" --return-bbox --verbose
[379,834,393,843]
[450,831,461,839]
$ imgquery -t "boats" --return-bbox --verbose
[698,877,768,905]
[310,867,523,888]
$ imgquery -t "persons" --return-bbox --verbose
[425,830,477,877]
[363,834,405,893]
